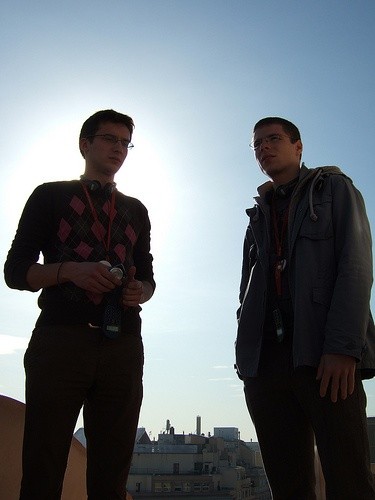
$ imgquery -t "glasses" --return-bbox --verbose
[249,134,292,150]
[87,134,134,150]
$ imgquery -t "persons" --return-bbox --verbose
[234,118,375,500]
[4,109,157,500]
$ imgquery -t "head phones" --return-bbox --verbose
[80,175,117,195]
[264,174,300,208]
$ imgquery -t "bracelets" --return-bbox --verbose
[56,262,66,282]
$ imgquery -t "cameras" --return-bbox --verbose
[106,263,129,283]
